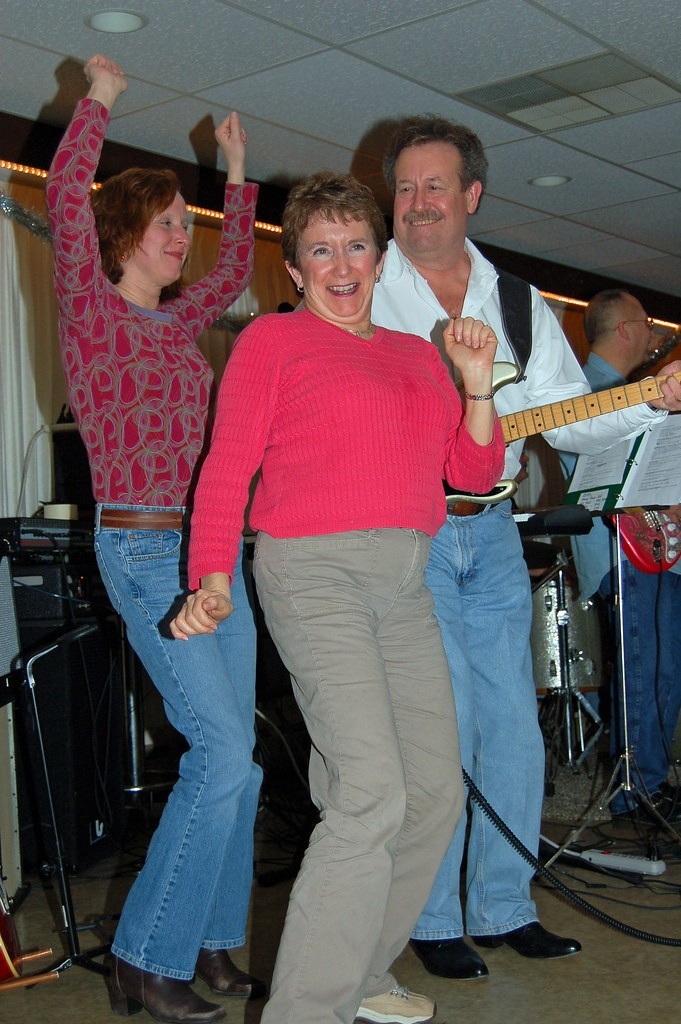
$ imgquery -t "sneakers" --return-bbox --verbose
[358,985,438,1024]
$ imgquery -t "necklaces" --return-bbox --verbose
[341,320,373,338]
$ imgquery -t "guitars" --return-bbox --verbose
[441,361,681,504]
[601,503,681,574]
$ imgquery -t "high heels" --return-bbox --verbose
[102,952,228,1023]
[197,946,254,997]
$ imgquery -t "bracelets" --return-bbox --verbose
[464,388,495,400]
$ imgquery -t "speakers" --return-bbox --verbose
[13,621,144,875]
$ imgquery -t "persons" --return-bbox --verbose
[367,113,681,983]
[169,170,507,1024]
[47,54,258,1024]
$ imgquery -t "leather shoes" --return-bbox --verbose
[472,922,582,962]
[408,936,491,982]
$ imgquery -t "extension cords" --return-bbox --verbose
[579,849,666,875]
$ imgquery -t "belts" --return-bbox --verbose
[446,491,503,517]
[93,506,185,531]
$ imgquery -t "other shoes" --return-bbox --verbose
[610,783,681,821]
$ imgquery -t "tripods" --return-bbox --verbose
[22,622,109,992]
[538,410,681,870]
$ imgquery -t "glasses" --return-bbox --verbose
[612,317,655,332]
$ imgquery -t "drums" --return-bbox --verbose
[529,568,605,696]
